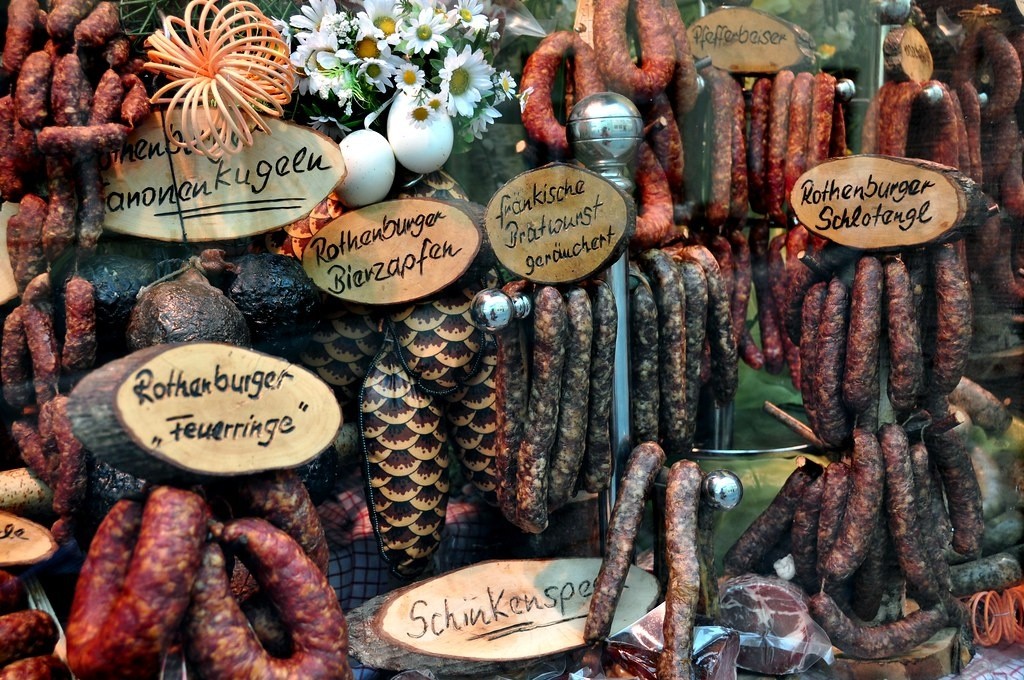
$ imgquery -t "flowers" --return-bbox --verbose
[268,0,518,149]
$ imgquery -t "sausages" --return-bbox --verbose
[0,0,1024,680]
